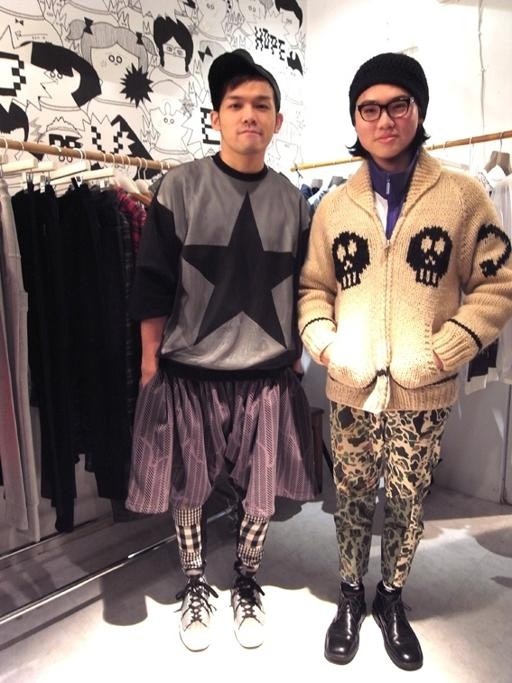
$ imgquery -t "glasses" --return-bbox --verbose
[354,95,414,122]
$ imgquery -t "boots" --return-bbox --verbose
[179,565,214,651]
[229,565,268,649]
[372,578,423,672]
[325,580,367,664]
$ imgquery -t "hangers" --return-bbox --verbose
[483,131,511,180]
[0,137,155,207]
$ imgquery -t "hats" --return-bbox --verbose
[207,53,282,113]
[349,53,429,128]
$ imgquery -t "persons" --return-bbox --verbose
[297,52,512,670]
[124,47,323,653]
